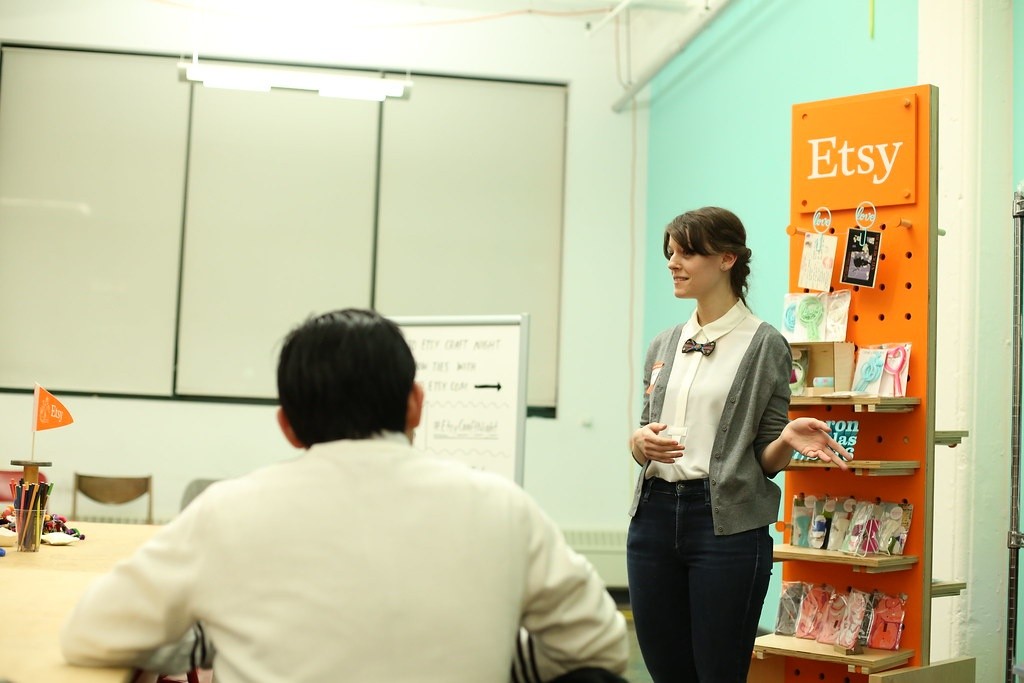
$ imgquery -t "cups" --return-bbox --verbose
[14,509,47,552]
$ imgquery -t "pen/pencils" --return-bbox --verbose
[9,479,55,553]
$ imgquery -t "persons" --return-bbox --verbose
[62,308,630,683]
[626,205,853,682]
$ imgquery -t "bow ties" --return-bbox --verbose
[682,339,716,356]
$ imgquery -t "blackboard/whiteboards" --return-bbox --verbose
[386,312,531,490]
